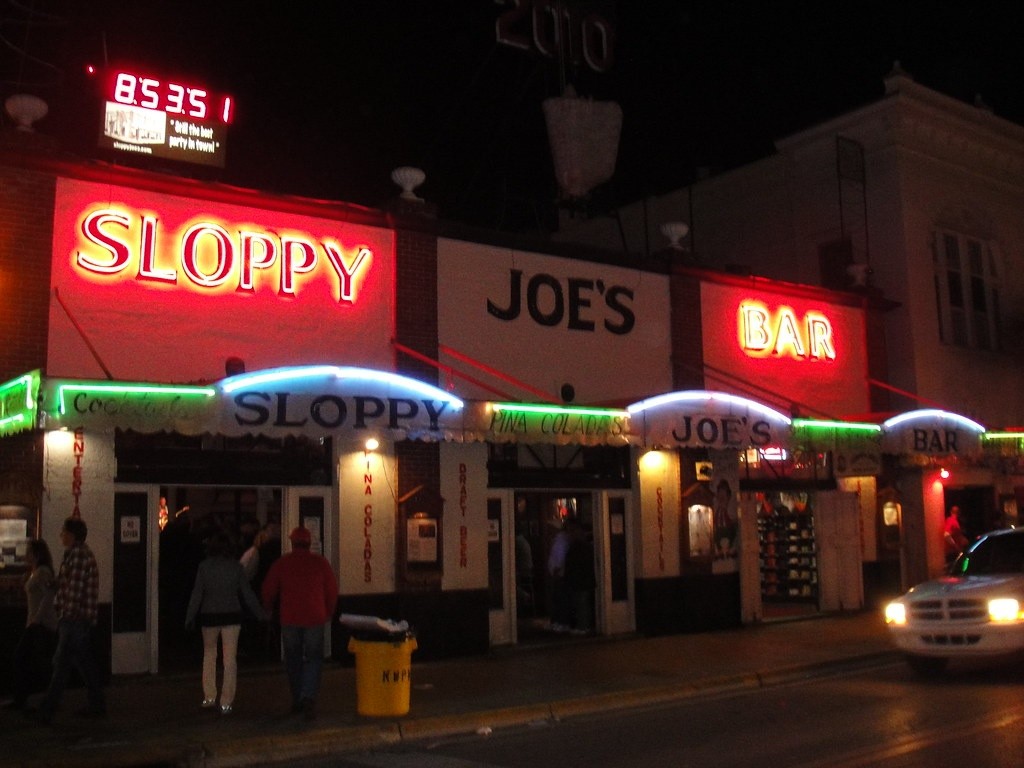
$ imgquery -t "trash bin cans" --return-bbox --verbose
[340,614,418,718]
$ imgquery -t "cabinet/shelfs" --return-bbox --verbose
[755,513,821,604]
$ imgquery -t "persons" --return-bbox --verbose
[22,534,55,724]
[550,520,584,627]
[261,527,337,720]
[939,505,968,561]
[183,534,264,714]
[38,516,107,719]
[515,527,535,620]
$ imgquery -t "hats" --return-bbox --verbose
[288,526,312,545]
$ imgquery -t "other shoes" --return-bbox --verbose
[301,697,316,720]
[219,704,233,714]
[0,698,29,709]
[202,699,215,707]
[72,704,107,718]
[291,703,302,713]
[26,709,50,724]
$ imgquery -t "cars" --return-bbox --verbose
[881,525,1024,675]
